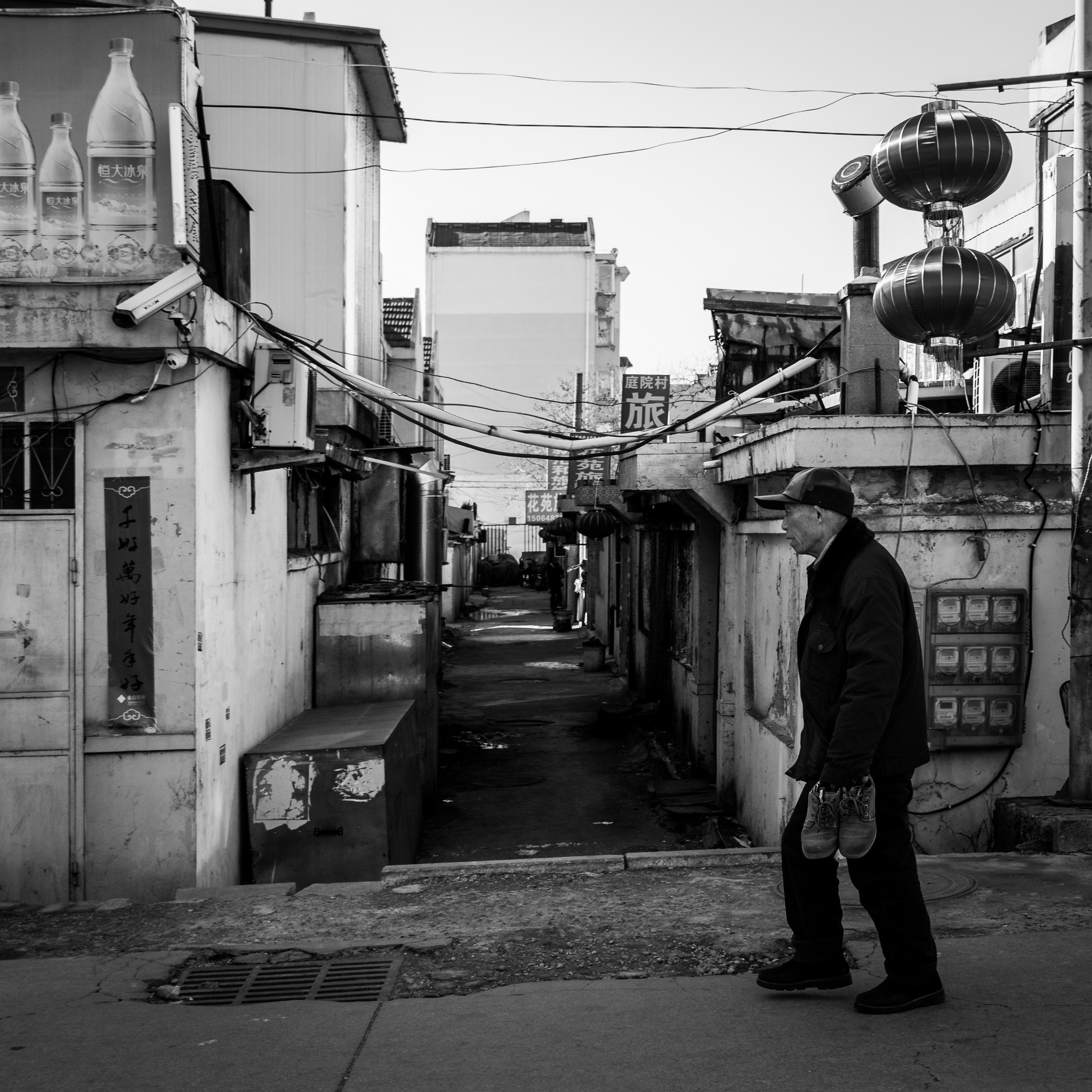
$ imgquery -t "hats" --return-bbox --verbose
[753,468,854,515]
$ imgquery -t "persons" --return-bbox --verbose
[580,559,588,626]
[547,555,565,616]
[754,467,945,1014]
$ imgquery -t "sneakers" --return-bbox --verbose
[756,947,853,991]
[855,969,944,1015]
[801,779,841,860]
[838,774,877,859]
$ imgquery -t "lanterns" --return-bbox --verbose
[577,509,616,541]
[539,516,574,538]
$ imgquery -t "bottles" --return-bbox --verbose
[89,36,157,276]
[40,113,84,277]
[0,81,37,278]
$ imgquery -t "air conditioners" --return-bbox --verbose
[972,354,1042,416]
[252,343,320,452]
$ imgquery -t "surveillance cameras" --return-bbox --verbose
[111,263,203,328]
[164,349,189,370]
